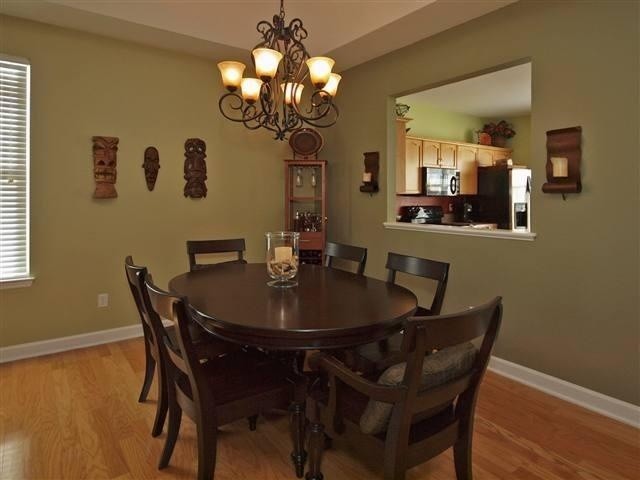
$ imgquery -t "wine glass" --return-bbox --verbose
[263,230,300,289]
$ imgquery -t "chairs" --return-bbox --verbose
[321,251,450,404]
[185,239,245,273]
[124,256,230,402]
[313,293,502,477]
[303,239,367,377]
[137,275,300,479]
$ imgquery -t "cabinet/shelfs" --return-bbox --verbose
[395,118,528,195]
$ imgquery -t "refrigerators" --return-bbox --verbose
[477,166,531,234]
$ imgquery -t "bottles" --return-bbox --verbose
[293,208,301,231]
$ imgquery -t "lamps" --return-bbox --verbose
[217,0,342,142]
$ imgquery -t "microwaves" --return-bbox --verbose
[422,167,460,197]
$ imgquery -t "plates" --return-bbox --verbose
[288,128,325,157]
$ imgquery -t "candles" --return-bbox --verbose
[274,245,295,266]
[362,172,372,183]
[550,157,568,178]
